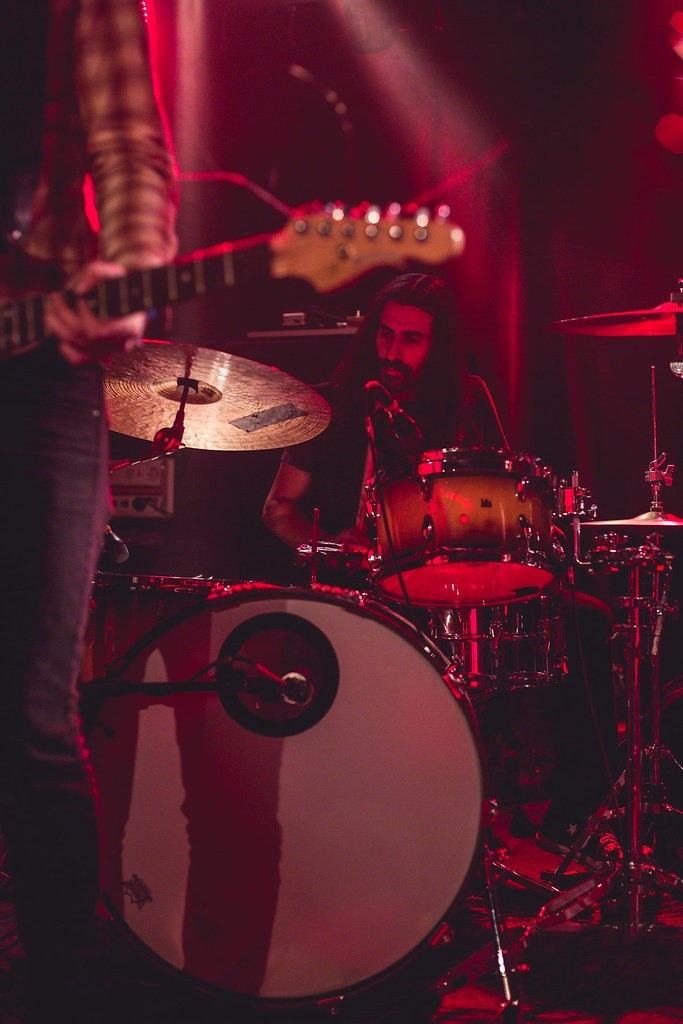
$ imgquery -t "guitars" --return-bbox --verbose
[1,199,467,362]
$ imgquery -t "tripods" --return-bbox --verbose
[491,527,683,983]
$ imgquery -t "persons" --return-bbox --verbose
[0,0,182,1024]
[261,273,512,594]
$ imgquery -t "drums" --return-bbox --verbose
[428,598,572,692]
[358,444,562,605]
[80,588,488,1004]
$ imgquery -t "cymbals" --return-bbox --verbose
[101,338,332,453]
[546,298,682,339]
[576,508,680,528]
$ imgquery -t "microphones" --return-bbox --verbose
[364,380,422,448]
[256,664,314,706]
[102,524,129,562]
